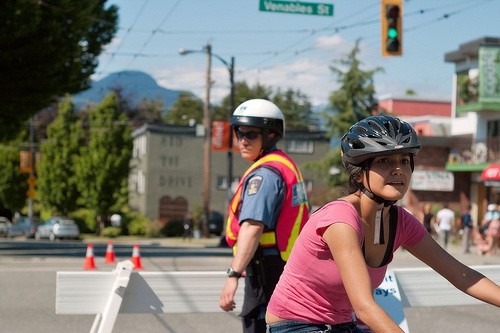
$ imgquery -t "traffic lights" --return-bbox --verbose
[386,4,400,53]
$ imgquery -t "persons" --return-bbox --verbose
[436,203,455,249]
[182,211,194,243]
[460,203,476,253]
[478,204,500,244]
[420,203,435,237]
[265,115,500,333]
[219,98,310,333]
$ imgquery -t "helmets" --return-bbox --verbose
[341,116,421,175]
[230,99,285,150]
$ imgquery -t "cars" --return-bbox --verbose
[7,217,46,238]
[0,216,13,237]
[36,217,80,241]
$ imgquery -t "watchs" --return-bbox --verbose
[227,268,241,278]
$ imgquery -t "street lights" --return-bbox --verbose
[177,46,237,244]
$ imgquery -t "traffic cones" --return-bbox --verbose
[130,244,144,270]
[102,241,117,264]
[82,243,96,271]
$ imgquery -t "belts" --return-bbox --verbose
[261,249,279,256]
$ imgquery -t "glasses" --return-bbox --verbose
[235,130,262,140]
